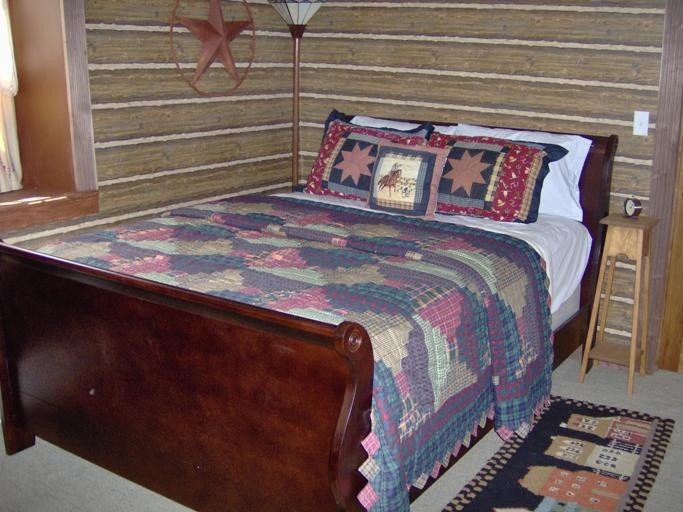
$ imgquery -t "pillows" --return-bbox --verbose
[427,131,569,224]
[454,122,592,222]
[303,109,435,203]
[363,141,451,221]
[350,114,456,135]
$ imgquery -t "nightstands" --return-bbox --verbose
[578,214,660,397]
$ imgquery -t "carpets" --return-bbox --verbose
[441,394,675,512]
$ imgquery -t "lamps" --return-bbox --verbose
[268,0,328,193]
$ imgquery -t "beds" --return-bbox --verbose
[0,109,619,512]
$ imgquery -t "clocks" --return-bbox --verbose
[623,198,642,220]
